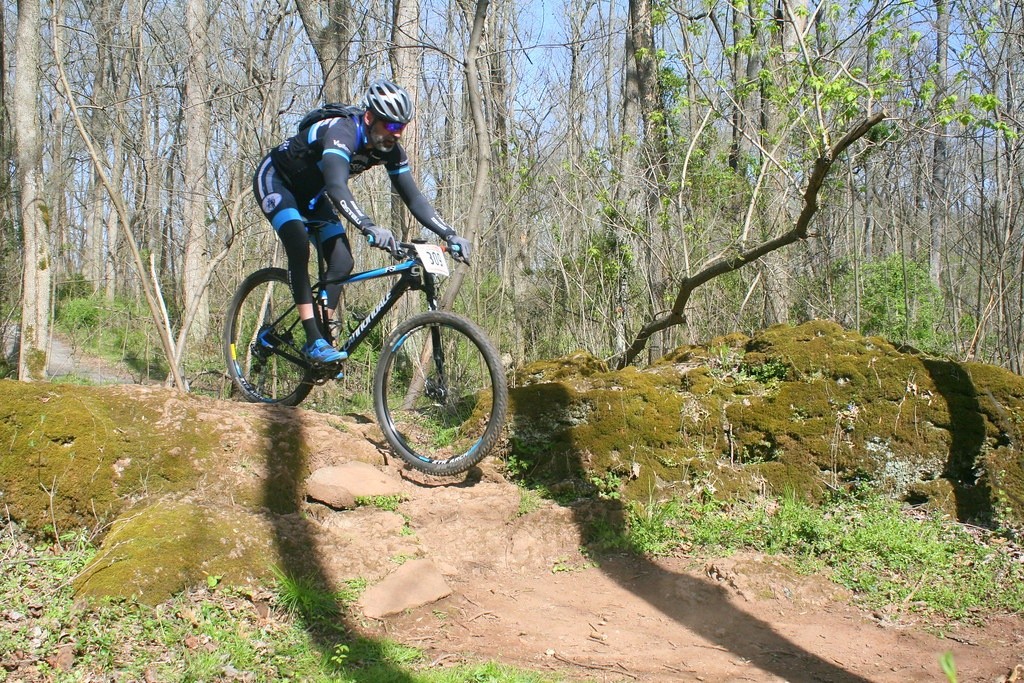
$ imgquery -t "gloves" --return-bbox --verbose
[448,235,471,265]
[361,224,398,256]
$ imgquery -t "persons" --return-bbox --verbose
[253,79,472,383]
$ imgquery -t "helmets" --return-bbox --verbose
[366,79,413,124]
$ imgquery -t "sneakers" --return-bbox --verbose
[306,339,348,365]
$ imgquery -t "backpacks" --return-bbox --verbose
[299,103,366,131]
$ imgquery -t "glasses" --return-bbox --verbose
[378,117,406,133]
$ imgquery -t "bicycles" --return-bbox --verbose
[220,230,508,477]
